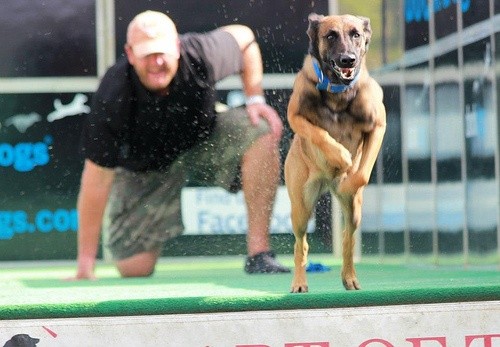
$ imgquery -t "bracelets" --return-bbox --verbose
[245,95,265,106]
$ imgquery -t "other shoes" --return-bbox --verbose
[245,251,290,273]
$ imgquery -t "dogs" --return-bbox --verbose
[285,12,387,293]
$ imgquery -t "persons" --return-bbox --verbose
[61,10,291,282]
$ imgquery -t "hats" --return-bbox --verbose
[127,8,179,60]
